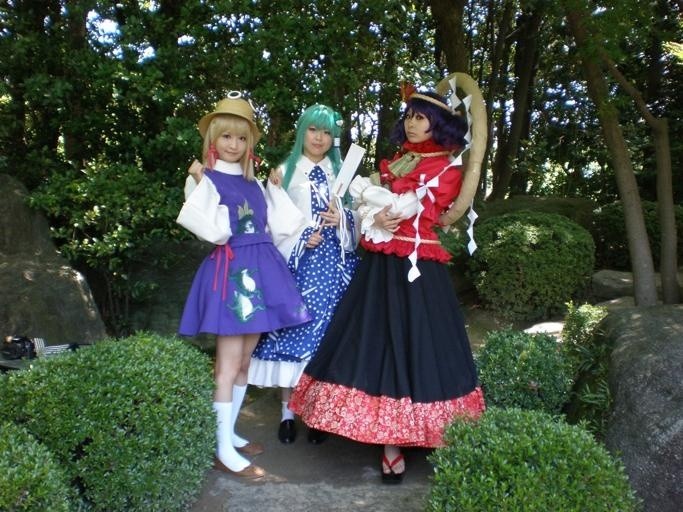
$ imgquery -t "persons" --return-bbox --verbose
[287,91,488,487]
[246,103,364,446]
[175,90,313,482]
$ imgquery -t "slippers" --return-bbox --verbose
[380,450,407,485]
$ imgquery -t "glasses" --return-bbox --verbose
[225,90,261,114]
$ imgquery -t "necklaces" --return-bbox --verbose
[386,150,450,179]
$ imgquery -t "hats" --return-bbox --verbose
[197,95,263,148]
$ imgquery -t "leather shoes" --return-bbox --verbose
[233,440,263,456]
[212,455,265,479]
[277,418,297,444]
[307,427,329,444]
